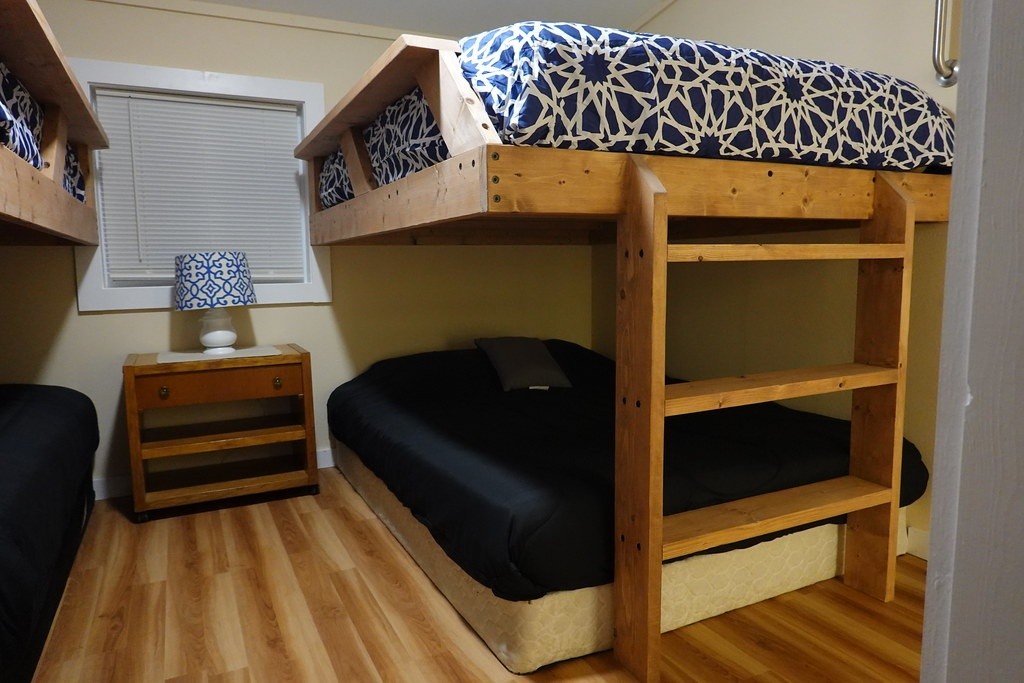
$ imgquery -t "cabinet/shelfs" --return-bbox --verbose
[123,344,320,522]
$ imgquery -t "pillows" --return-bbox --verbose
[475,337,573,392]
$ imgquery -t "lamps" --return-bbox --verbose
[175,251,260,355]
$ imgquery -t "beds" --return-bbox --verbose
[0,0,110,682]
[293,20,955,674]
